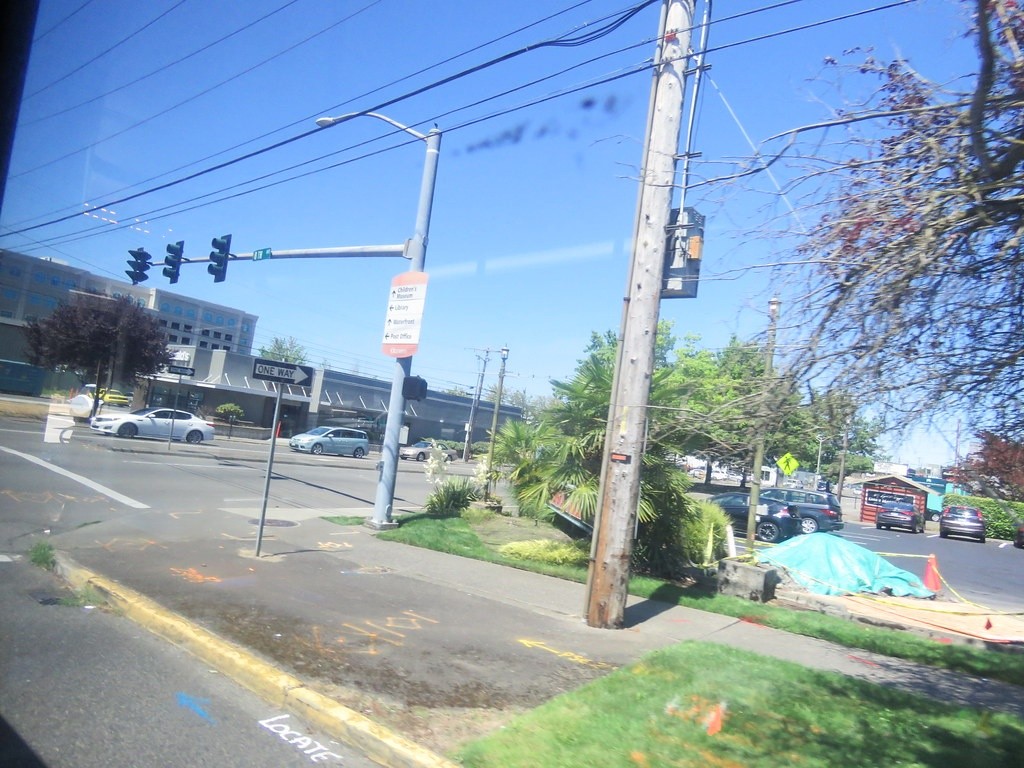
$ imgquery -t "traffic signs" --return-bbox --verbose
[251,357,315,389]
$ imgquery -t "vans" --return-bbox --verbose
[288,425,371,458]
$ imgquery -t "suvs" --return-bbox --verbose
[756,488,844,534]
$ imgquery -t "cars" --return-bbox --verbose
[398,441,458,462]
[84,383,162,408]
[88,404,216,445]
[1013,521,1024,546]
[927,507,941,522]
[939,505,988,543]
[875,500,927,534]
[703,491,802,544]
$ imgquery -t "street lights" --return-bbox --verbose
[484,342,510,500]
[314,110,442,532]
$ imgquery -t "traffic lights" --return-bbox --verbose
[125,247,152,285]
[206,233,233,283]
[162,240,184,284]
[401,377,428,402]
